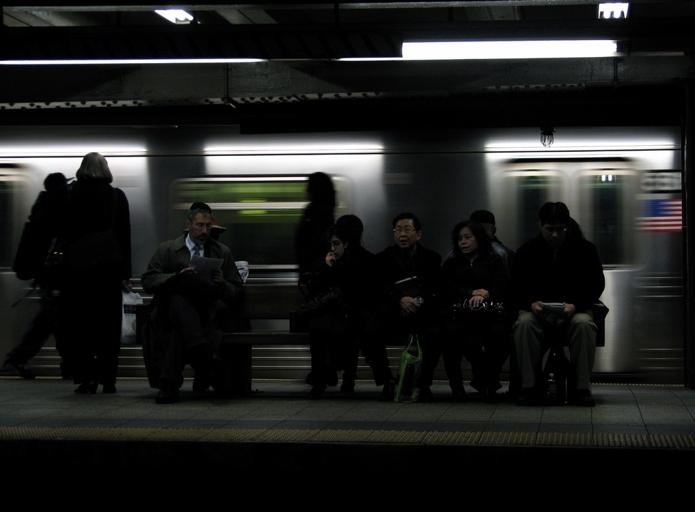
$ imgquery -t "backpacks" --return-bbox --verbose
[11,191,59,280]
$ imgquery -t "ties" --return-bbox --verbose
[191,241,204,261]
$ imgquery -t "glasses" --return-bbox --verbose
[392,225,416,234]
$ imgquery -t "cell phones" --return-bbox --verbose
[413,296,425,305]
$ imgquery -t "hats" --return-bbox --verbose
[45,173,70,190]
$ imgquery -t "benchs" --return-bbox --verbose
[122,281,610,407]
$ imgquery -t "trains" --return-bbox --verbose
[0,124,695,384]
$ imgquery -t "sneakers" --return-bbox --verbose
[2,355,37,378]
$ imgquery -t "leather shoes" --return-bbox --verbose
[152,382,212,406]
[304,368,595,408]
[73,375,119,394]
